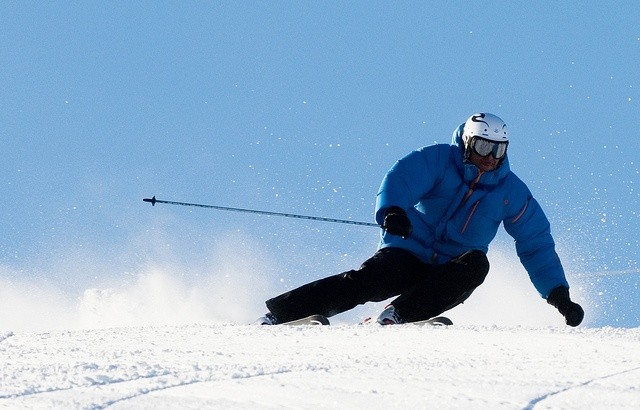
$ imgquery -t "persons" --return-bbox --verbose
[252,112,584,327]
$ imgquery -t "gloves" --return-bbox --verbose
[547,286,584,327]
[383,206,410,239]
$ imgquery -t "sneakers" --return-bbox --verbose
[376,302,405,328]
[254,313,276,325]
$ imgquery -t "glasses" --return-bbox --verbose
[469,136,508,159]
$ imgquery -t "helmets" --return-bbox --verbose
[461,113,508,149]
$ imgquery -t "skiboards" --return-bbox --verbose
[279,314,453,325]
